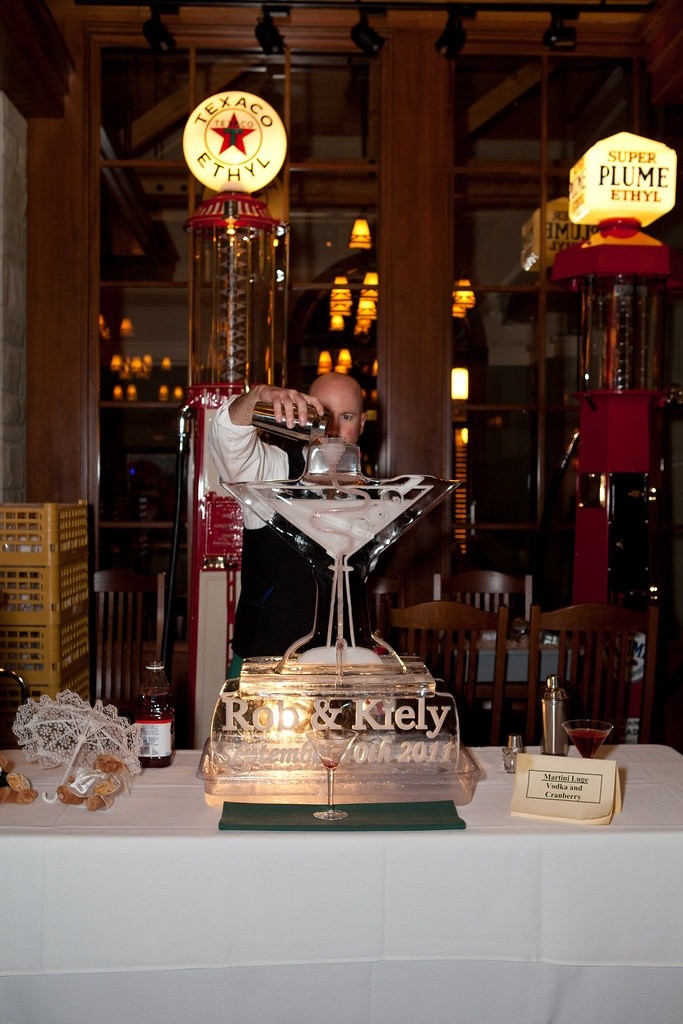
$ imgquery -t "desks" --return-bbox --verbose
[0,746,682,1024]
[452,646,571,684]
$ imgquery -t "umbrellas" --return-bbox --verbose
[13,687,143,806]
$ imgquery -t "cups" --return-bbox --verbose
[251,401,329,442]
[562,719,614,758]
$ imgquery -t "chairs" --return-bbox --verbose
[364,569,658,745]
[91,570,168,713]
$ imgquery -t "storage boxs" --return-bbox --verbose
[0,499,91,749]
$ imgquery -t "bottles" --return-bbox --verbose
[132,660,176,769]
[503,735,527,773]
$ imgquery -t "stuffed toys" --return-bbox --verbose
[0,755,36,804]
[56,754,124,811]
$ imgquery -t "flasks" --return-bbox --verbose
[540,672,570,760]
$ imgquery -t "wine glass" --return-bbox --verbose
[305,727,357,822]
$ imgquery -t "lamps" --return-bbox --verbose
[435,10,477,59]
[256,6,291,55]
[142,4,179,55]
[543,10,580,52]
[350,8,387,57]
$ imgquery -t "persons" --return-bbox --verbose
[210,372,367,685]
